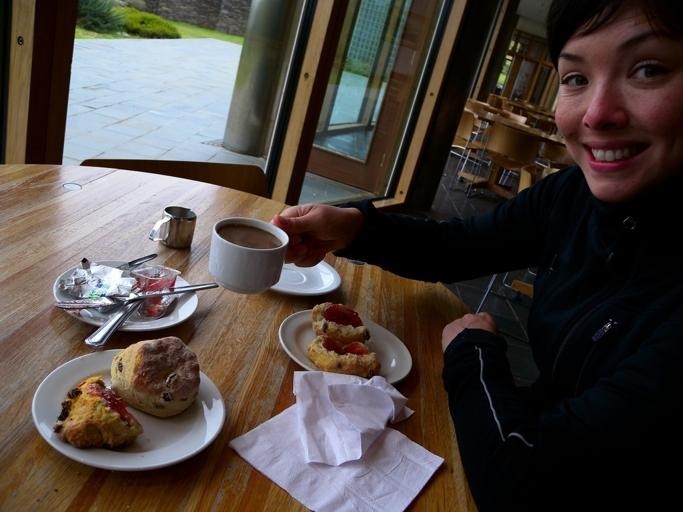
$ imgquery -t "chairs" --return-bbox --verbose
[446,84,576,314]
[80,154,266,196]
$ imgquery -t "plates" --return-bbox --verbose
[277,309,415,384]
[30,348,227,472]
[266,257,342,297]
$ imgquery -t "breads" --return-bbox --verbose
[57,375,143,450]
[113,336,201,418]
[309,336,380,377]
[312,302,370,345]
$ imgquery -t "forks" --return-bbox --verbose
[53,260,198,334]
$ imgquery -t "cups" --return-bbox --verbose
[148,205,196,250]
[204,216,288,296]
[130,264,182,319]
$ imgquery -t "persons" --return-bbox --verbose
[268,0,682,510]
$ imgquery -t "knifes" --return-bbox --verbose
[55,282,218,310]
